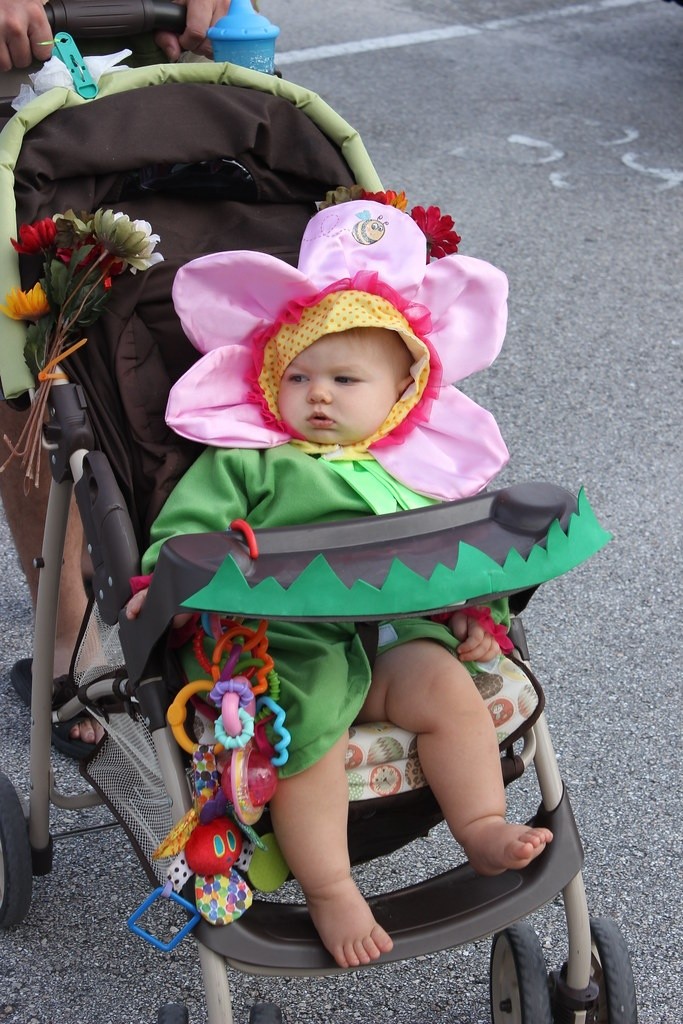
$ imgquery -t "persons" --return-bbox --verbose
[0,0,261,758]
[128,309,557,970]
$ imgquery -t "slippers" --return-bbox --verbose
[7,657,108,762]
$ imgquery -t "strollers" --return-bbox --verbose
[0,1,639,1024]
